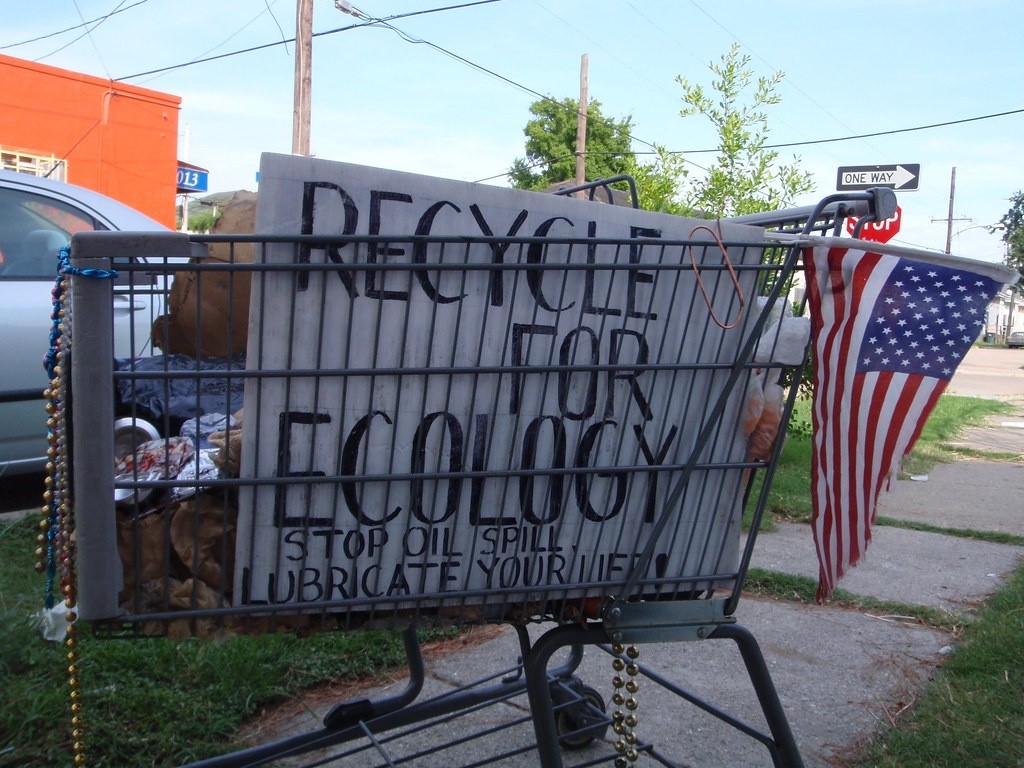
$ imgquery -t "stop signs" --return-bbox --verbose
[848,204,902,244]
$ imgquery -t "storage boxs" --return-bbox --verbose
[148,190,255,360]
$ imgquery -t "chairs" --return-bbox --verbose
[1,229,67,277]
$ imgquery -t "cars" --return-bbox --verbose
[1006,331,1024,348]
[1,169,224,480]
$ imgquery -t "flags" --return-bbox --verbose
[801,237,1018,603]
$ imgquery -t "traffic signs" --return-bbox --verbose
[836,163,923,194]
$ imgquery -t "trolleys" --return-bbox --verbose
[39,194,810,768]
